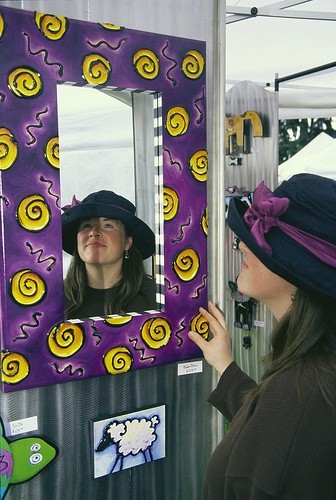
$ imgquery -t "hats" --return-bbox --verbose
[61,190,156,260]
[228,173,336,299]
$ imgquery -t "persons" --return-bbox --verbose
[188,172,336,500]
[61,190,157,320]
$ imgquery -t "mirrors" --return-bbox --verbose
[56,80,161,321]
